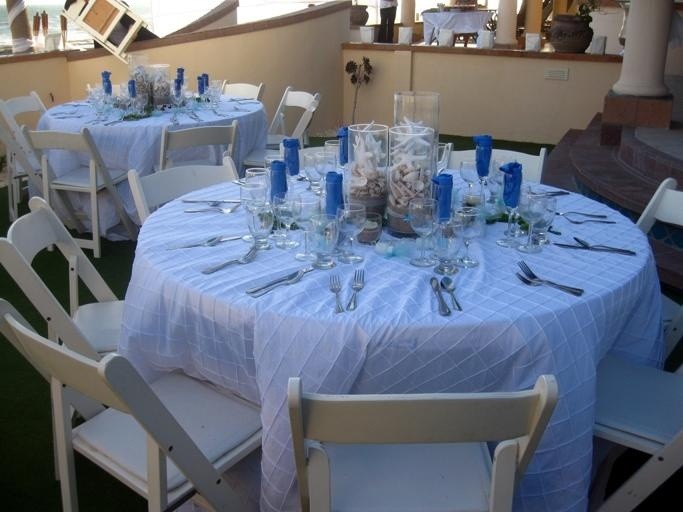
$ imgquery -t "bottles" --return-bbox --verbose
[348,91,441,236]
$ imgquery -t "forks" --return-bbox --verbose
[202,246,255,274]
[515,273,584,293]
[544,206,616,224]
[183,205,240,216]
[346,269,364,310]
[517,261,581,297]
[328,275,344,313]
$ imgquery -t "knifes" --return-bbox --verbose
[430,277,451,315]
[245,265,312,294]
[529,187,568,200]
[553,242,633,255]
[193,235,243,245]
[182,199,242,203]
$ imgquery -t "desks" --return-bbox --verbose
[120,164,660,508]
[423,9,494,48]
[37,97,268,240]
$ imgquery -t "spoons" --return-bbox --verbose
[439,276,462,311]
[574,237,635,254]
[165,234,222,249]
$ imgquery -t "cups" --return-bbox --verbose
[397,26,413,44]
[438,28,455,47]
[476,30,494,50]
[590,35,606,55]
[525,34,541,53]
[360,26,375,44]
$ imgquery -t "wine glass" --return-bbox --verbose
[244,129,367,270]
[457,137,502,210]
[502,161,555,253]
[50,54,256,128]
[408,193,485,274]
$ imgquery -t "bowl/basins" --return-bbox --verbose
[349,5,367,26]
[548,15,594,51]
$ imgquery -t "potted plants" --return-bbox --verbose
[543,0,598,54]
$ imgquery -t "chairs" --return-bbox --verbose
[288,374,558,510]
[2,197,126,479]
[0,297,264,510]
[0,59,322,260]
[441,142,545,185]
[592,348,683,509]
